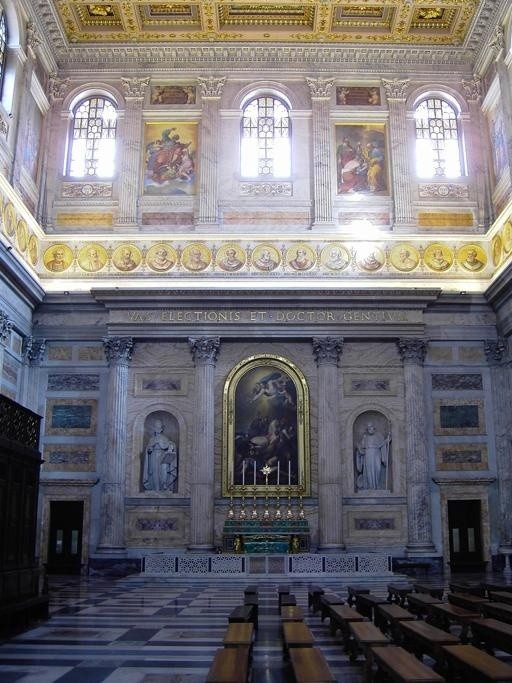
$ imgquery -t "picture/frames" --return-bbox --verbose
[227,358,305,493]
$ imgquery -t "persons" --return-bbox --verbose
[141,418,178,492]
[236,372,297,484]
[355,423,392,490]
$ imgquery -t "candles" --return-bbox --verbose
[230,461,233,486]
[277,461,280,485]
[300,460,302,484]
[243,460,245,485]
[254,460,256,485]
[289,461,291,485]
